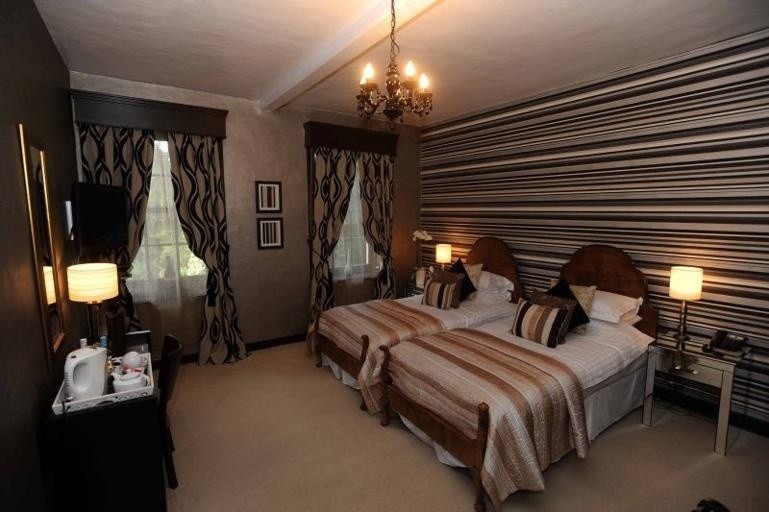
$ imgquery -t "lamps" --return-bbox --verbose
[61,256,119,343]
[356,1,436,120]
[664,265,704,343]
[434,242,452,274]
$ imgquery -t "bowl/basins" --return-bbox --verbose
[128,344,149,354]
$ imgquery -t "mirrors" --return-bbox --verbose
[17,120,69,369]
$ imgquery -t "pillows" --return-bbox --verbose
[422,258,518,309]
[506,276,646,349]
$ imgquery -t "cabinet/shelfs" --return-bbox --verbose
[35,335,170,512]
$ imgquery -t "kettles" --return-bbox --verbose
[63,346,107,400]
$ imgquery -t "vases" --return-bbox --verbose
[415,266,426,290]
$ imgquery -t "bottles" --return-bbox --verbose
[99,335,113,373]
[78,338,89,349]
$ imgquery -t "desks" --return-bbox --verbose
[404,282,423,297]
[642,331,751,458]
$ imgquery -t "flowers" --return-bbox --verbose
[409,229,432,266]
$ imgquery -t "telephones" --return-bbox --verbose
[710,330,748,351]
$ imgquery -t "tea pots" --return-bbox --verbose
[110,368,151,393]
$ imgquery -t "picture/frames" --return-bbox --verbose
[253,178,283,215]
[256,216,283,252]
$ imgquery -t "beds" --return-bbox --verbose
[359,243,659,511]
[305,234,522,410]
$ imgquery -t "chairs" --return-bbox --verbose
[152,334,180,495]
